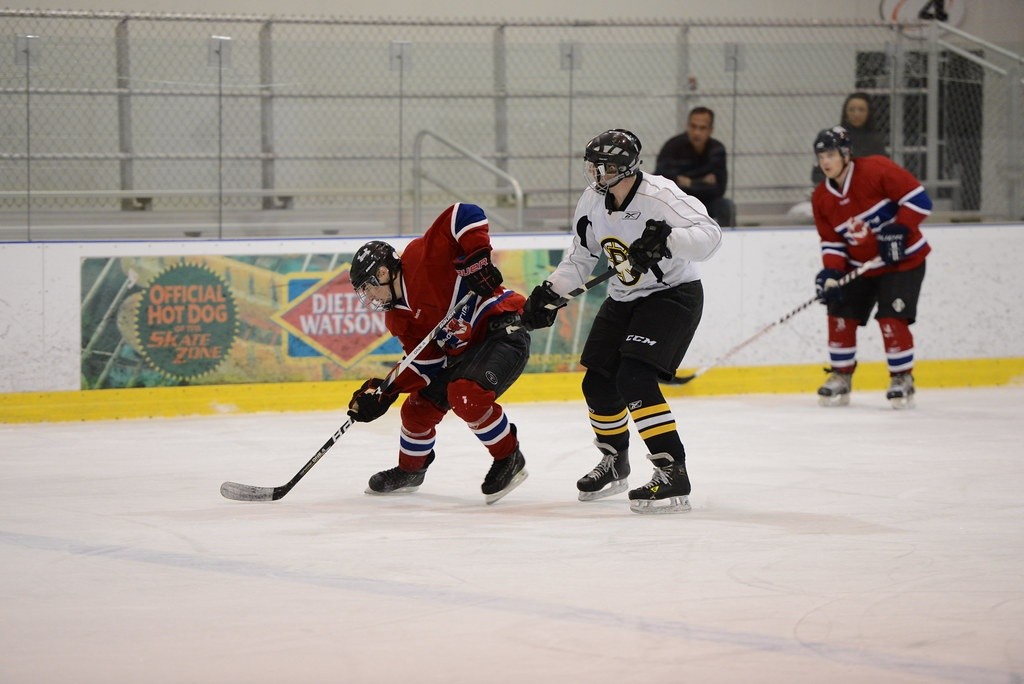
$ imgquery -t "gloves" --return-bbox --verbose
[520,280,567,332]
[630,218,672,271]
[347,378,400,422]
[874,221,909,266]
[453,247,503,298]
[815,266,846,306]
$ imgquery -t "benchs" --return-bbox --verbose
[0,179,982,238]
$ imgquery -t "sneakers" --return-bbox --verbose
[886,371,915,408]
[364,449,435,495]
[482,423,528,504]
[628,452,692,514]
[577,437,631,501]
[817,361,858,405]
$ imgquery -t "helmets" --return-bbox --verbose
[349,241,401,289]
[583,129,642,179]
[814,126,852,155]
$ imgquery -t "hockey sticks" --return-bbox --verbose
[220,288,477,501]
[663,252,891,386]
[488,256,638,341]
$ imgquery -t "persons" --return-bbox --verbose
[652,107,736,226]
[347,203,531,505]
[523,128,724,513]
[840,92,890,157]
[812,126,933,409]
